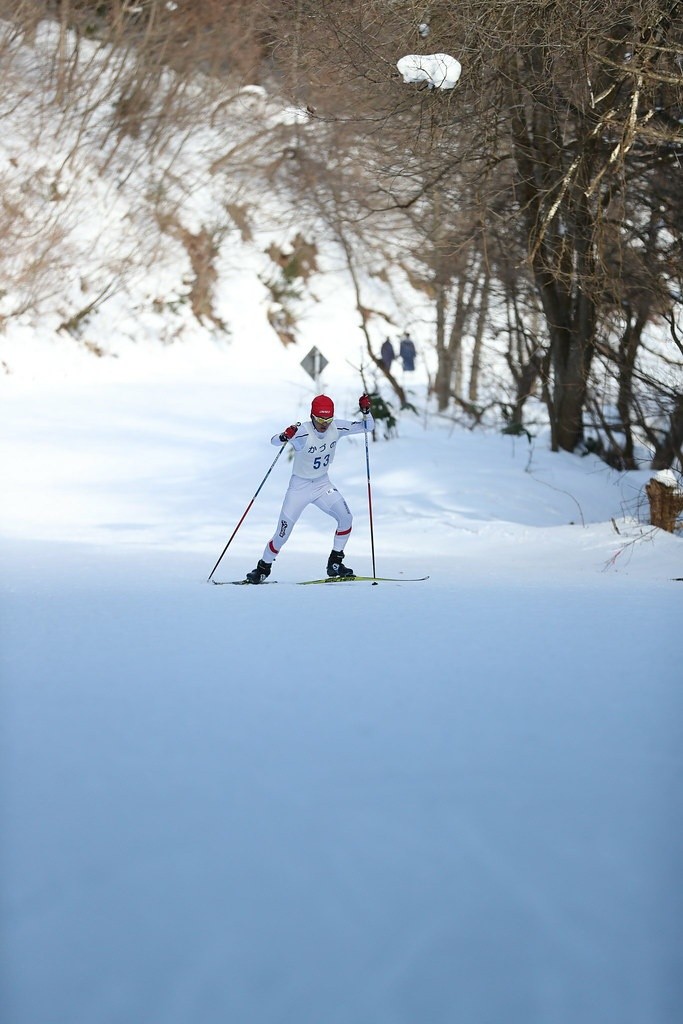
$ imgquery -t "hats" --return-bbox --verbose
[311,395,334,417]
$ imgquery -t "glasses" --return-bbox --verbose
[311,414,333,424]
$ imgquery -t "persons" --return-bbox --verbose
[381,332,416,374]
[246,394,375,584]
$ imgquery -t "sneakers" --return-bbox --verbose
[246,560,272,584]
[326,550,353,576]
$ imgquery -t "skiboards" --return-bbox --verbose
[211,575,431,585]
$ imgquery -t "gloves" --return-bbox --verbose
[359,393,370,414]
[279,425,298,442]
